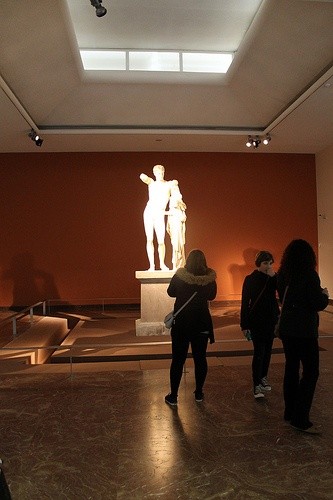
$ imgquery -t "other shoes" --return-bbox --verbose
[195,393,203,402]
[289,423,321,434]
[165,394,178,405]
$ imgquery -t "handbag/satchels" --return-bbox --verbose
[274,311,284,337]
[164,311,175,328]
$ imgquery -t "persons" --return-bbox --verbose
[140,165,186,270]
[277,238,329,433]
[165,250,218,405]
[240,250,280,399]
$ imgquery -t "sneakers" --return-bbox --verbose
[253,385,264,398]
[260,378,271,391]
